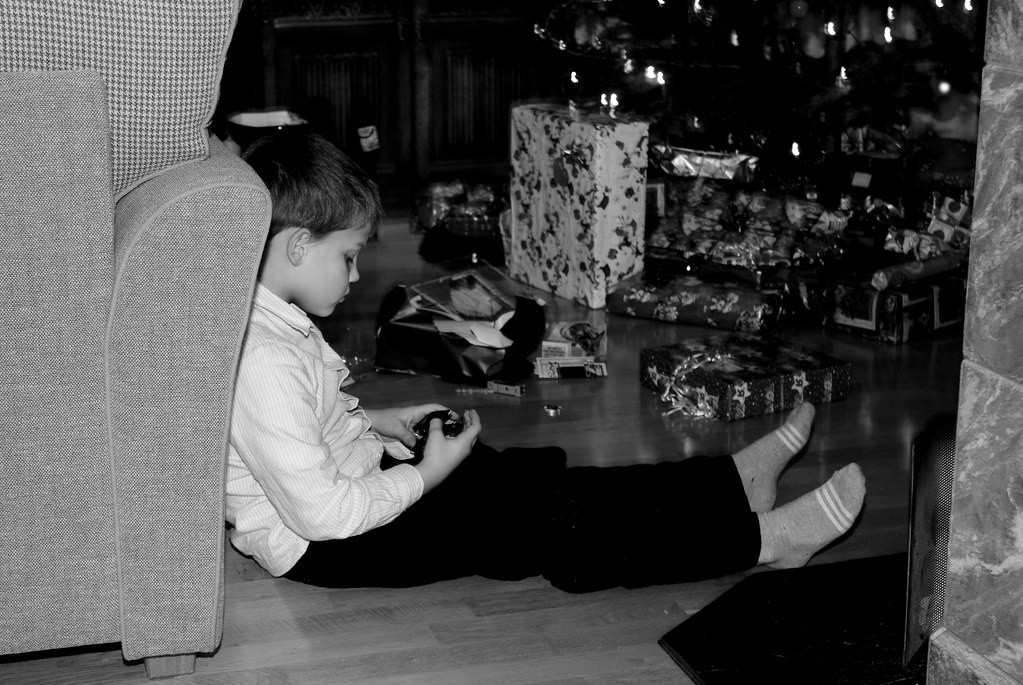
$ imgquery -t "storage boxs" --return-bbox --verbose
[509,104,651,307]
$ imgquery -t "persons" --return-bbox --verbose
[224,126,867,594]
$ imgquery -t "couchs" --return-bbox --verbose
[0,0,271,679]
